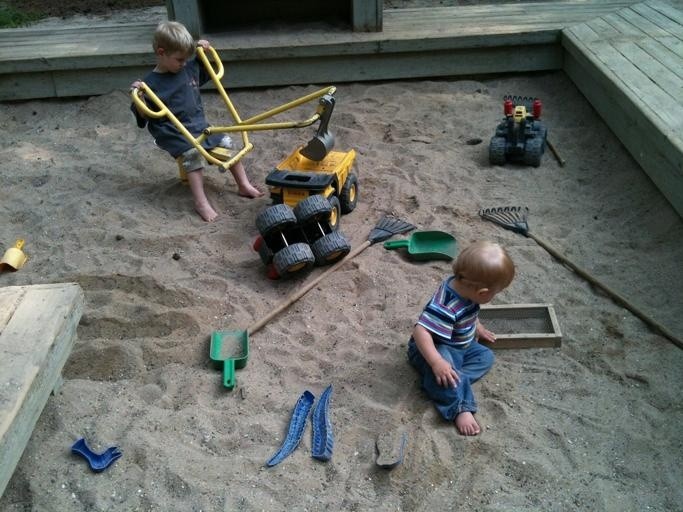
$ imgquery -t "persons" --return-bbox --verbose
[406,236,517,437]
[127,22,265,222]
